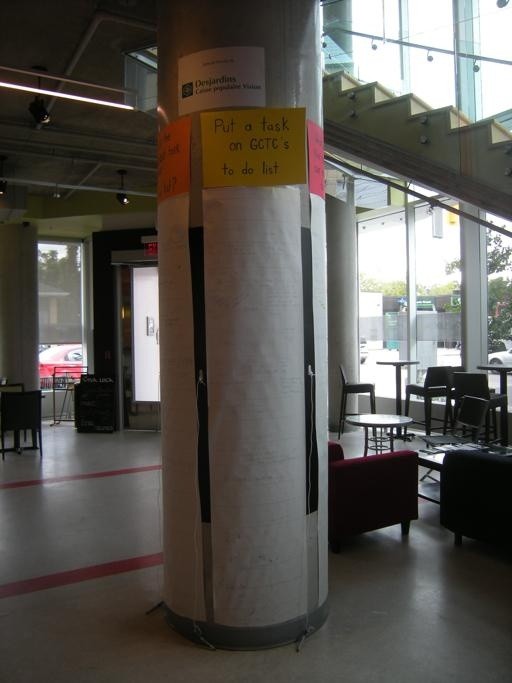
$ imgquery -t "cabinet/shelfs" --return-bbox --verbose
[73,374,129,434]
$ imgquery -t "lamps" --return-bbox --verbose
[48,182,62,200]
[320,29,330,49]
[472,57,483,71]
[29,66,52,124]
[426,49,434,61]
[370,38,378,51]
[116,169,130,206]
[0,180,9,200]
[497,0,509,8]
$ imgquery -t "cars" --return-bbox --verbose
[488,347,512,374]
[39,344,83,386]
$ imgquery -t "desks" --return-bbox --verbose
[344,410,511,507]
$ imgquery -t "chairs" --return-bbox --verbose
[439,448,511,548]
[1,382,43,462]
[329,442,419,556]
[337,360,512,445]
